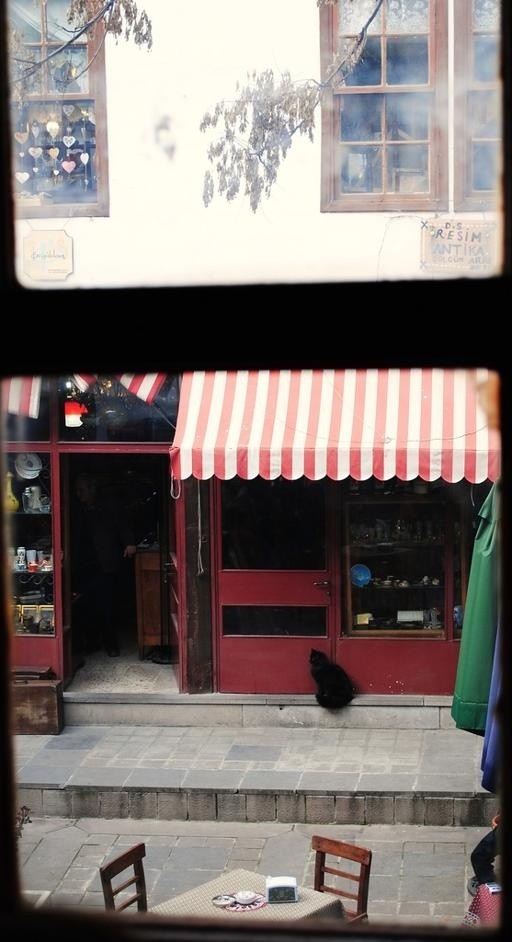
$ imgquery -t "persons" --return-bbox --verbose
[466,824,502,897]
[71,473,138,657]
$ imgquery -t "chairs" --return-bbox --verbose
[311,836,371,927]
[100,843,148,916]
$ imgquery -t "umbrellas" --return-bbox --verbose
[449,476,500,737]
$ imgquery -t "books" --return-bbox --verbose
[267,885,299,902]
[484,880,503,895]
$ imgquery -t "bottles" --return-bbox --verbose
[22,488,34,512]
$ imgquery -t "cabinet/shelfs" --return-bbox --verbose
[0,440,73,690]
[341,495,468,638]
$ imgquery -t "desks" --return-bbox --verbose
[459,882,501,928]
[129,549,163,662]
[147,869,346,921]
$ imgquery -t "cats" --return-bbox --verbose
[308,648,355,710]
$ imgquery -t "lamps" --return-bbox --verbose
[64,398,89,427]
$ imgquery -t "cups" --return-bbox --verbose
[29,564,38,572]
[16,564,25,571]
[26,550,36,563]
[236,891,255,904]
[45,567,52,570]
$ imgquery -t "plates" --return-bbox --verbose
[40,569,53,572]
[14,452,43,480]
[351,564,371,587]
